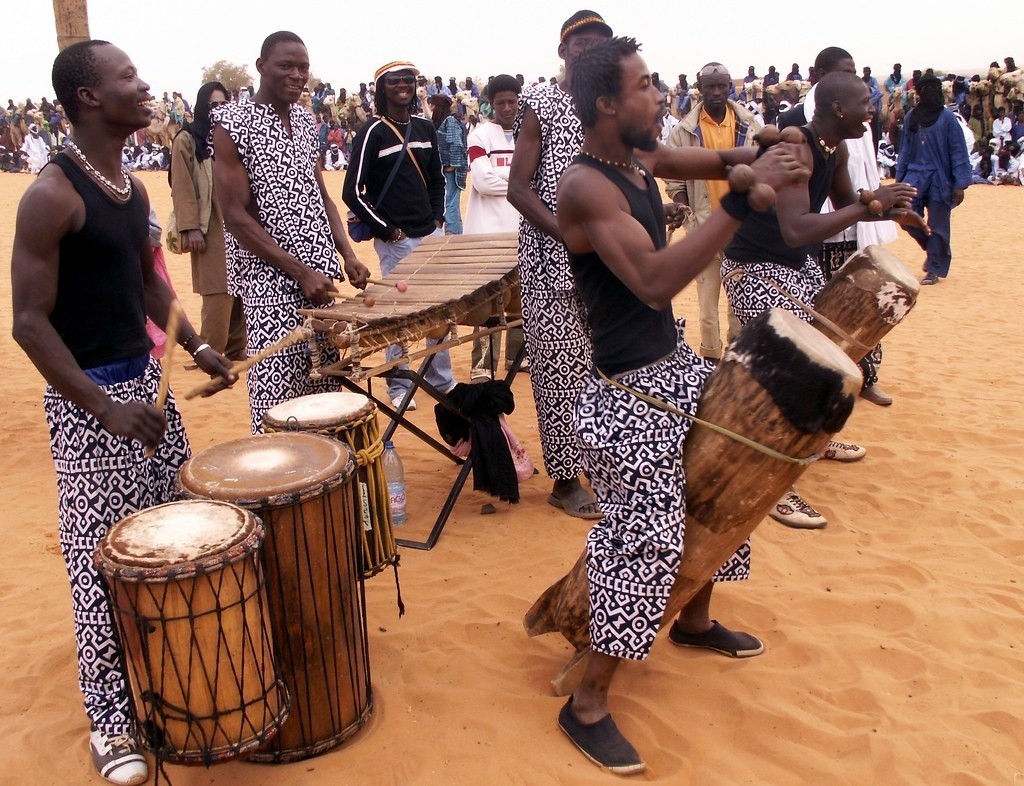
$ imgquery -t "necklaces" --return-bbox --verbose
[578,150,646,176]
[384,114,410,124]
[812,122,837,154]
[67,142,132,202]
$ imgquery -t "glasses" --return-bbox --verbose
[209,100,228,108]
[385,75,417,85]
[698,65,728,77]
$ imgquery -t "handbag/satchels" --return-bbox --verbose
[346,195,379,243]
[165,210,190,255]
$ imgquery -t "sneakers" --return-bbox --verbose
[820,440,866,461]
[392,392,416,410]
[443,382,458,395]
[769,485,828,529]
[88,722,148,786]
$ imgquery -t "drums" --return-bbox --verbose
[259,393,401,580]
[524,308,864,697]
[806,244,921,363]
[174,433,375,762]
[93,497,290,764]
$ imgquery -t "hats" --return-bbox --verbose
[560,9,613,46]
[374,60,419,84]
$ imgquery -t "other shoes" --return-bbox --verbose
[470,376,490,384]
[668,619,764,657]
[921,273,937,285]
[557,694,645,773]
[504,362,530,373]
[923,257,929,271]
[227,346,247,360]
[860,382,892,404]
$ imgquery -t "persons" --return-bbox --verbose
[504,10,972,527]
[229,85,255,103]
[551,35,810,776]
[209,32,371,458]
[295,81,375,171]
[170,80,253,370]
[0,92,195,179]
[12,39,240,786]
[343,60,561,411]
[959,57,1024,187]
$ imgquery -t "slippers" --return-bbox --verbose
[547,489,604,518]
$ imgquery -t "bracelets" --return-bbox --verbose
[390,228,401,245]
[192,343,211,365]
[183,333,195,351]
[886,201,911,218]
[755,125,802,163]
[857,188,883,220]
[719,164,776,225]
